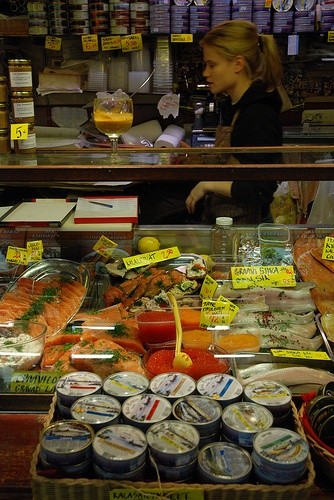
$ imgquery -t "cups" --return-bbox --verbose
[105,56,128,92]
[152,37,174,94]
[129,45,151,97]
[85,60,108,91]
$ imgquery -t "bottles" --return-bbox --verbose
[210,217,237,262]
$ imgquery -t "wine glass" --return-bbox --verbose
[92,96,135,164]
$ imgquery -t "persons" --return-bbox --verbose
[180,20,283,223]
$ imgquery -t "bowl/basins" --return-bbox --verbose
[0,320,48,374]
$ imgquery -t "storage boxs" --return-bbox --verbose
[0,19,29,37]
[39,73,84,88]
